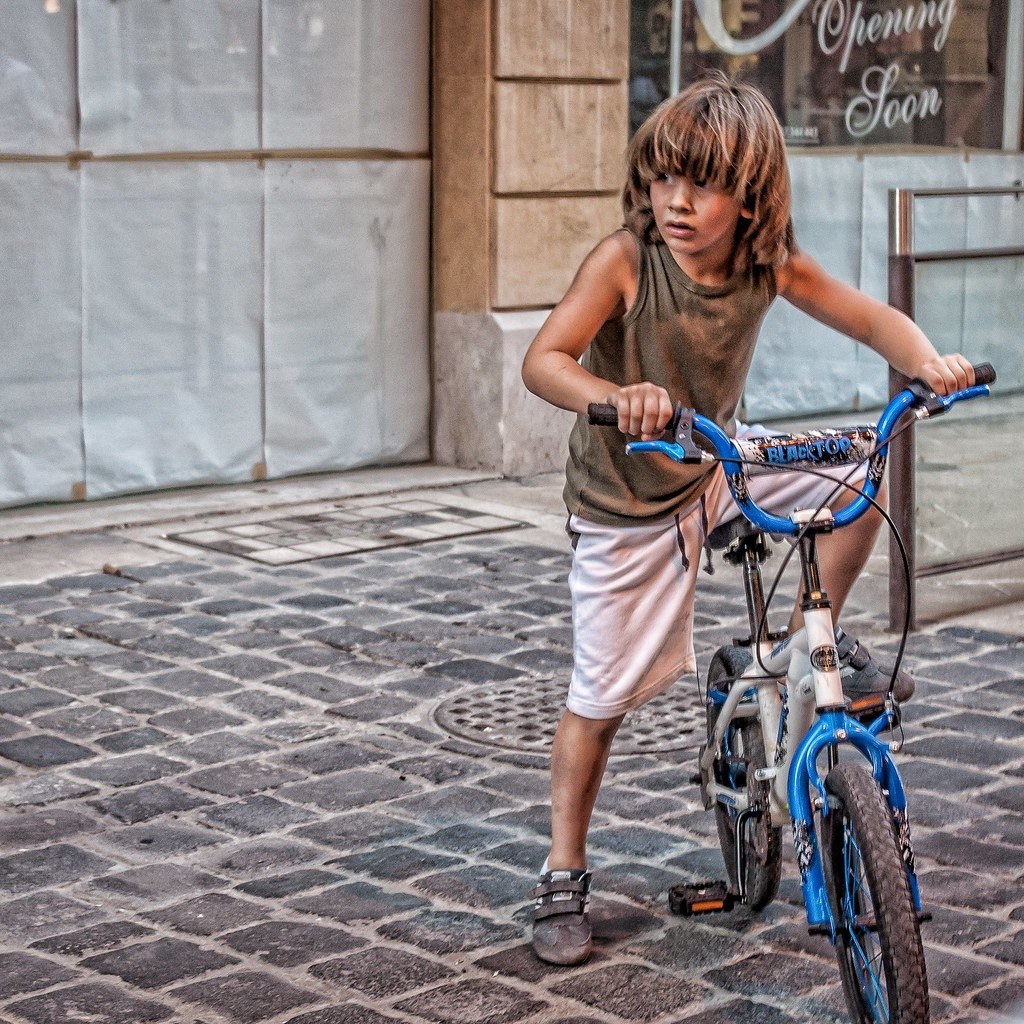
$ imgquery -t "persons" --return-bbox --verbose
[522,69,977,965]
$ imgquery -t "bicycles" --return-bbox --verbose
[587,359,998,1024]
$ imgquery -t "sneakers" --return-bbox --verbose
[774,626,915,711]
[527,854,593,964]
